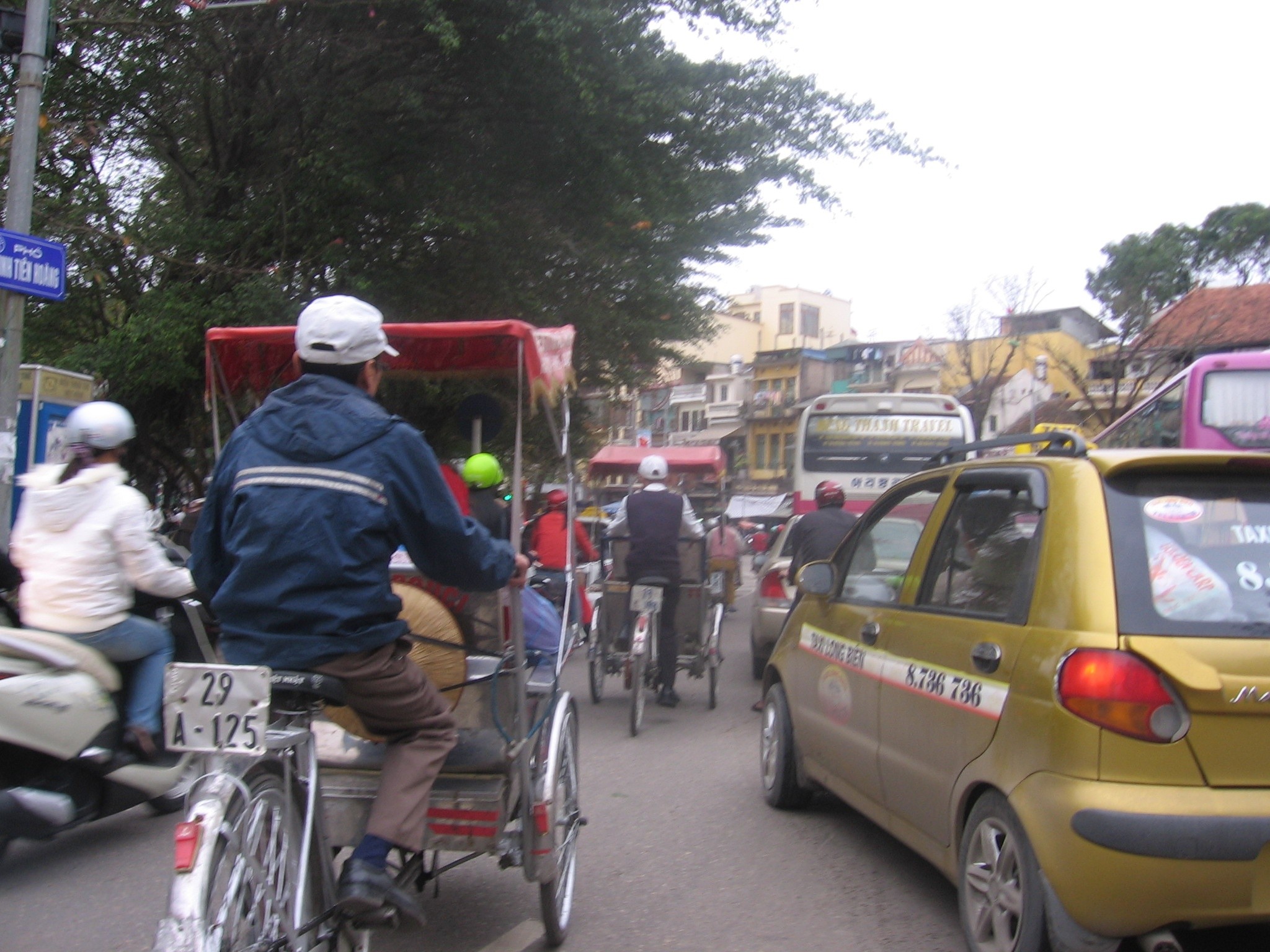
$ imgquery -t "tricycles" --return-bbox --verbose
[156,560,588,952]
[588,533,726,736]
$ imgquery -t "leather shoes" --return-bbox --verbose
[339,859,435,931]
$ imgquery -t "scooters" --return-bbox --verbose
[0,498,222,852]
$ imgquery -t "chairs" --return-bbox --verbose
[964,531,1028,611]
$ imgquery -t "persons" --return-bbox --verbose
[752,481,877,710]
[8,400,207,759]
[932,494,1012,604]
[191,294,528,929]
[706,513,785,612]
[605,454,705,707]
[465,452,599,645]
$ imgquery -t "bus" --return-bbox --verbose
[1087,350,1270,557]
[794,393,977,526]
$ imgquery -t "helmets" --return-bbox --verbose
[463,453,504,488]
[546,489,568,511]
[62,401,137,449]
[816,480,844,508]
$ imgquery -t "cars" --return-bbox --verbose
[752,513,954,680]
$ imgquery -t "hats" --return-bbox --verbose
[638,455,668,480]
[296,295,400,364]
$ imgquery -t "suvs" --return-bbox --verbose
[759,432,1270,952]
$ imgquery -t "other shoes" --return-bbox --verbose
[657,691,679,707]
[122,730,163,766]
